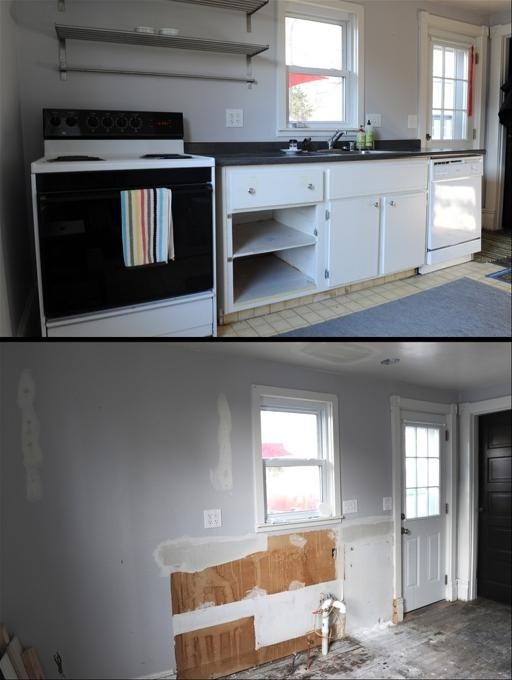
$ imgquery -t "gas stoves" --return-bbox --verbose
[30,152,215,174]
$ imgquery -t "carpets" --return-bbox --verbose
[474,228,512,285]
[271,275,512,338]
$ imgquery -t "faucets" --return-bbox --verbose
[328,128,347,149]
[312,598,348,656]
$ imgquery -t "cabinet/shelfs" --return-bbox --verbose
[328,159,428,288]
[225,164,325,310]
[53,0,270,90]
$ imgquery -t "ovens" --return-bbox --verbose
[35,166,214,320]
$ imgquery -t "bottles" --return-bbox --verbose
[365,120,375,149]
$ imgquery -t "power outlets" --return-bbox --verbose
[225,108,244,128]
[204,508,222,528]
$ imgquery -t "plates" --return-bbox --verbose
[280,148,302,155]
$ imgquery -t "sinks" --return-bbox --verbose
[314,147,370,155]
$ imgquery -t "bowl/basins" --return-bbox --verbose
[136,27,179,38]
